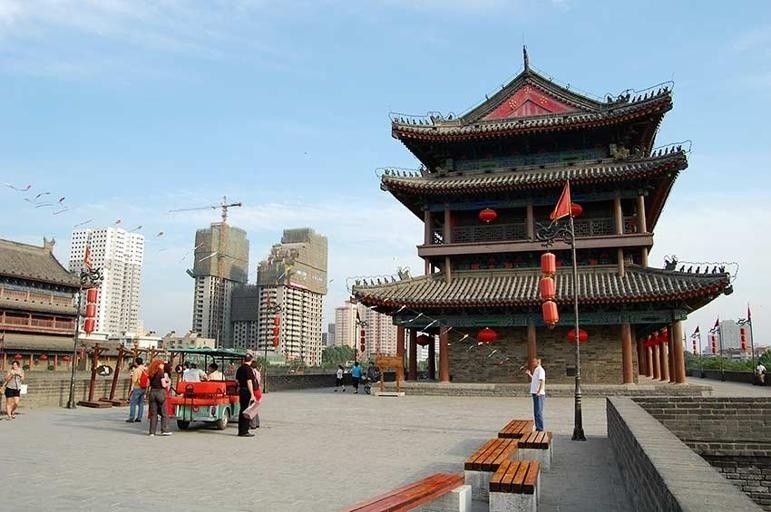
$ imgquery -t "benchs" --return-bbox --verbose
[336,471,472,511]
[465,418,554,512]
[171,380,239,405]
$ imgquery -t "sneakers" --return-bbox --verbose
[238,433,255,437]
[126,419,141,423]
[249,426,259,429]
[149,431,173,437]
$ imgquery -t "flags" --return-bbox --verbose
[545,180,571,229]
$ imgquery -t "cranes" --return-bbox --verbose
[167,194,242,348]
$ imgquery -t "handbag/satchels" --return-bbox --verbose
[0,384,6,395]
[19,385,27,395]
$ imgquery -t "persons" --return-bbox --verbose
[366,362,377,382]
[333,365,346,392]
[125,351,262,437]
[350,362,362,394]
[4,360,25,419]
[525,357,546,431]
[756,361,767,385]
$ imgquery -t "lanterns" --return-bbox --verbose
[14,353,22,361]
[643,328,668,348]
[84,286,97,336]
[541,251,560,328]
[740,327,746,351]
[693,340,697,356]
[479,207,498,223]
[39,354,48,361]
[273,311,280,347]
[712,335,716,353]
[62,355,70,362]
[566,329,589,345]
[417,333,429,346]
[360,329,365,352]
[477,328,498,344]
[569,201,583,216]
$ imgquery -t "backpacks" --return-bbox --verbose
[137,366,150,389]
